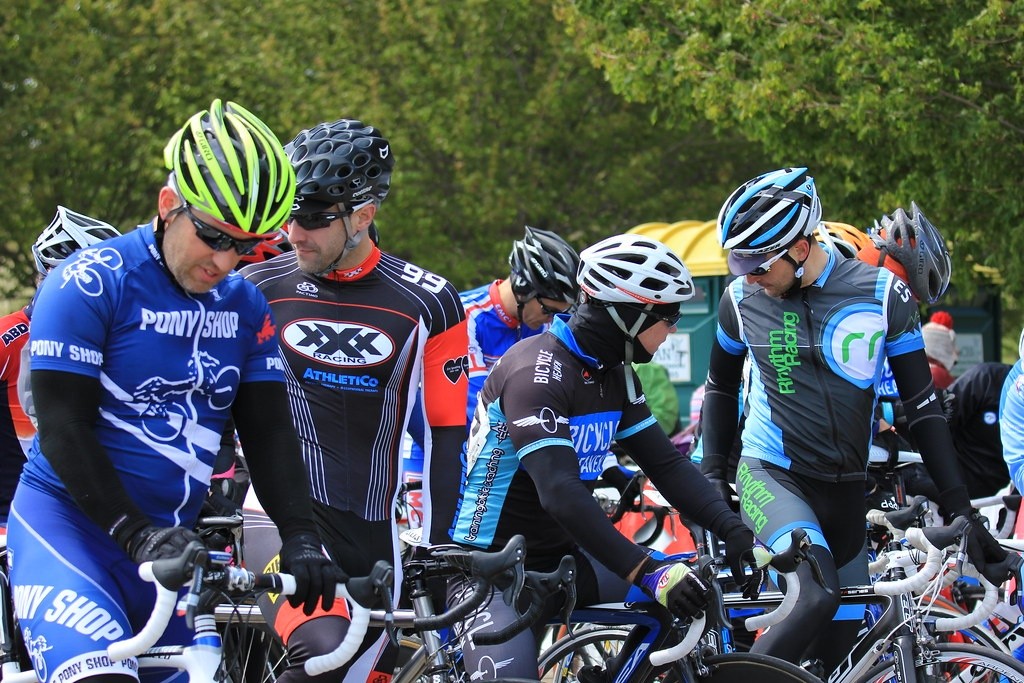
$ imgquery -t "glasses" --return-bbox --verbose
[173,174,265,256]
[620,303,681,328]
[285,198,375,230]
[536,296,569,317]
[750,233,803,276]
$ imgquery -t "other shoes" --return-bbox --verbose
[577,665,608,683]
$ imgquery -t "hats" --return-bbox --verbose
[727,249,766,276]
[921,311,959,371]
[290,157,377,215]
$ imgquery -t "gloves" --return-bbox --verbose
[129,526,211,577]
[935,485,1010,582]
[279,534,349,616]
[719,520,766,601]
[601,466,641,507]
[700,454,737,509]
[633,556,713,624]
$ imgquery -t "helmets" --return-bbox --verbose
[812,221,870,252]
[163,98,297,238]
[283,119,395,201]
[576,233,695,303]
[234,227,296,271]
[716,167,822,250]
[32,205,123,276]
[868,201,951,304]
[508,226,585,304]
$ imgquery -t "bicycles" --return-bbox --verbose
[0,460,1024,683]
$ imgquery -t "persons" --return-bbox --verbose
[0,99,1024,683]
